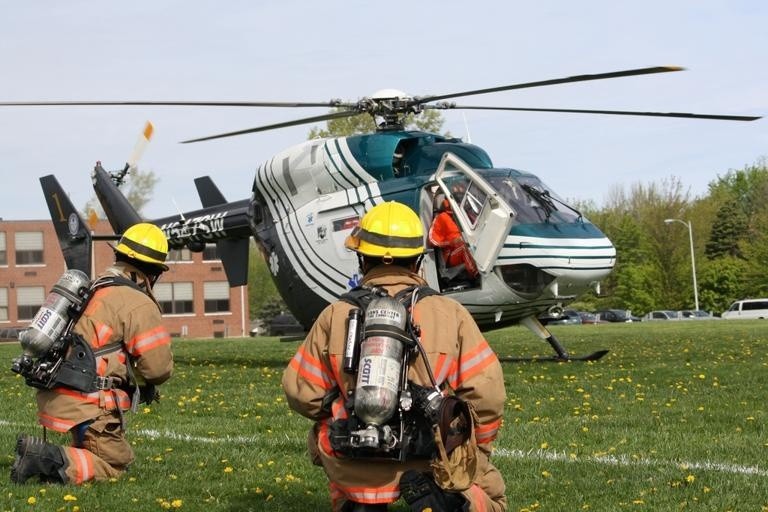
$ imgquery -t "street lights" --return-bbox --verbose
[663,219,699,311]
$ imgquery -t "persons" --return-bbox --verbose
[425,179,482,288]
[277,198,513,511]
[9,221,177,486]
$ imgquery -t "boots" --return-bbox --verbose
[10,434,63,485]
[399,469,471,511]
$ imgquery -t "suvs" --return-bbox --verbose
[268,313,306,336]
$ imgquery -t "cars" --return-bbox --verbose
[576,312,610,324]
[640,310,688,322]
[598,308,641,323]
[545,309,582,326]
[0,325,30,343]
[675,310,718,320]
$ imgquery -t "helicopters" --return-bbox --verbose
[0,66,762,363]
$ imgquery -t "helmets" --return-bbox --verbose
[106,223,169,272]
[344,201,434,259]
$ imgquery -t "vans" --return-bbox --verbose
[721,298,768,319]
